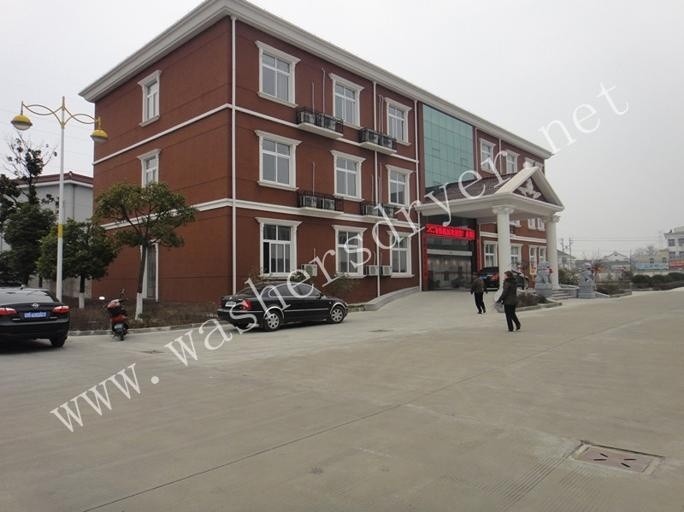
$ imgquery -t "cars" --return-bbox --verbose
[215,279,349,333]
[0,285,72,348]
[473,266,529,290]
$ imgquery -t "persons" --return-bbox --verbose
[495,271,520,332]
[470,272,488,314]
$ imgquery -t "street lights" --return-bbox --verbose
[10,94,110,304]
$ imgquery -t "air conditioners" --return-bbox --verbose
[361,204,394,218]
[366,264,392,278]
[362,131,393,149]
[297,111,336,133]
[300,263,318,278]
[298,194,336,211]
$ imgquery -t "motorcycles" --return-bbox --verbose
[98,288,129,341]
